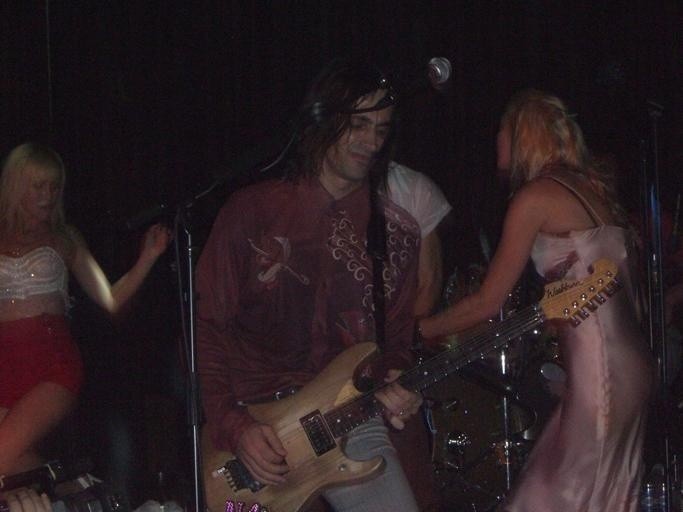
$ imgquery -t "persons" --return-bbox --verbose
[0,138,178,497]
[181,41,429,510]
[381,85,662,512]
[342,153,455,331]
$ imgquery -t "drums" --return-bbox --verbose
[414,345,541,509]
[437,273,541,368]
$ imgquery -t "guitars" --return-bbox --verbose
[201,258,623,512]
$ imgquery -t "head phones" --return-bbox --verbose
[297,96,339,139]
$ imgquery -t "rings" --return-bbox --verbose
[396,409,406,416]
